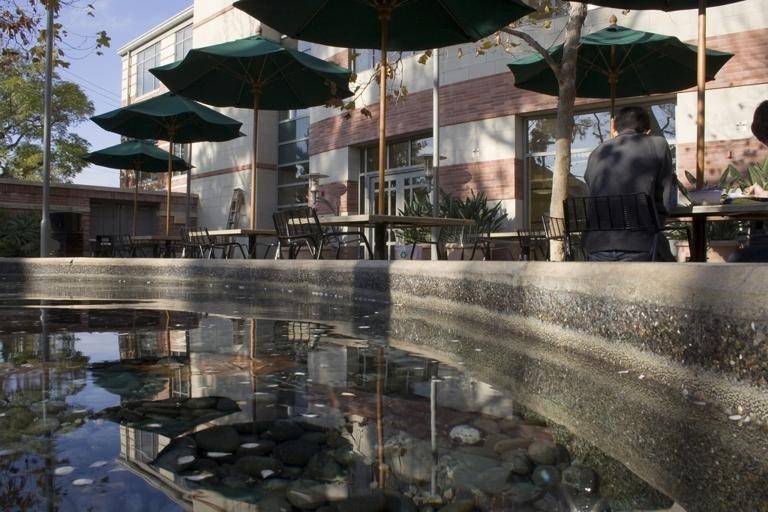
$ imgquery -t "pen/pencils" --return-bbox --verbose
[747,182,757,196]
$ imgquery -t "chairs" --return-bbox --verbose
[518,229,545,260]
[92,236,112,257]
[542,215,587,260]
[179,226,247,259]
[271,206,374,259]
[560,193,674,259]
[111,234,151,259]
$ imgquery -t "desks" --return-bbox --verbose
[672,203,768,262]
[188,227,278,260]
[92,234,182,257]
[287,213,476,261]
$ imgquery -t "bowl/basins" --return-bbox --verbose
[687,190,721,204]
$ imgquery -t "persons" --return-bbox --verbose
[581,106,677,261]
[749,101,766,145]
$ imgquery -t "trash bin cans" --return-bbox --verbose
[96,234,124,258]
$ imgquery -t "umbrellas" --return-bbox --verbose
[571,0,746,263]
[89,92,247,235]
[232,0,537,260]
[504,15,734,138]
[83,138,191,234]
[149,36,354,230]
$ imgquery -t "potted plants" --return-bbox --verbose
[664,166,753,262]
